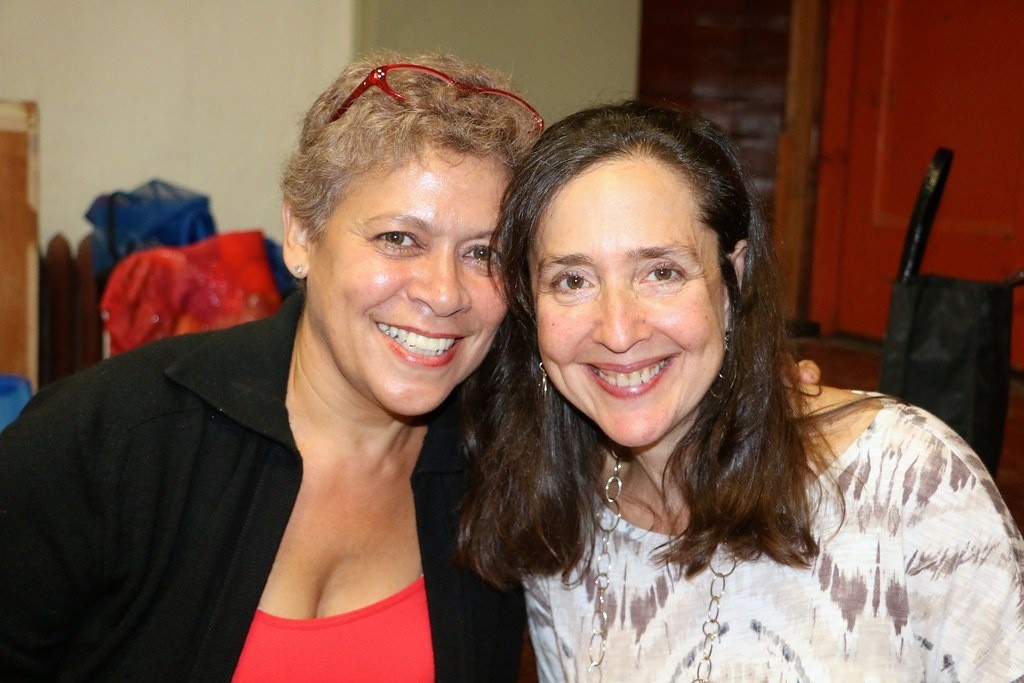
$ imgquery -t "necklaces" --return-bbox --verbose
[584,448,740,683]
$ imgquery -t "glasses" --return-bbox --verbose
[325,64,544,154]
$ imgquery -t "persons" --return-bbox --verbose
[453,99,1024,682]
[0,48,546,683]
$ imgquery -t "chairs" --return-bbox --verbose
[880,147,1024,479]
